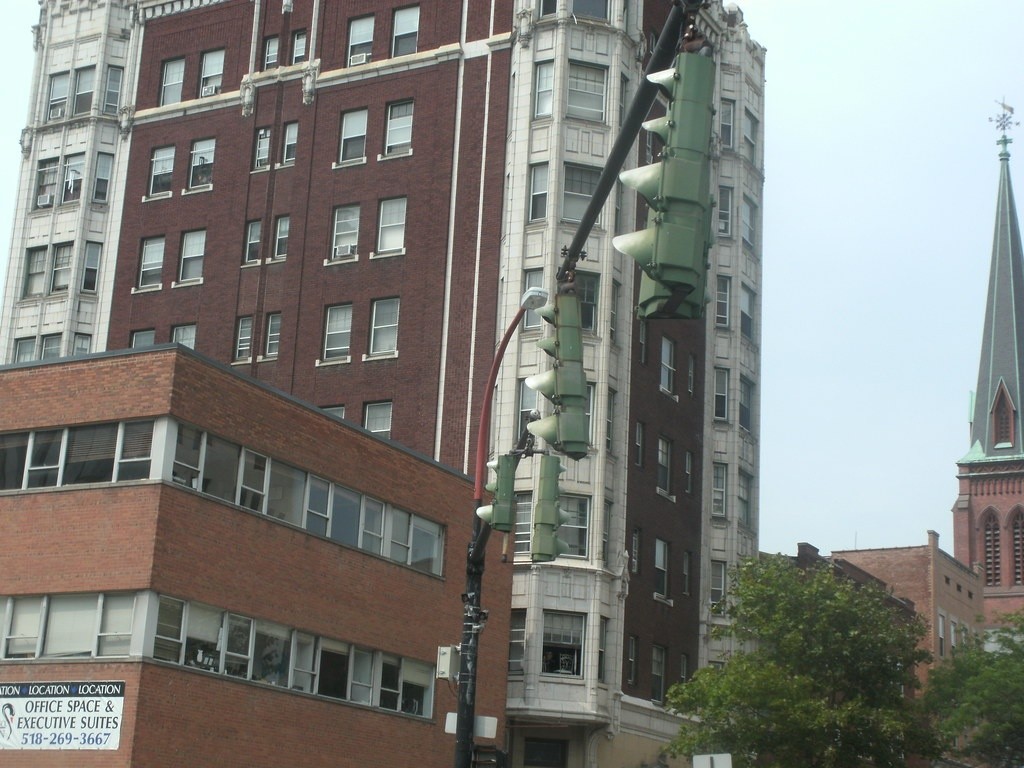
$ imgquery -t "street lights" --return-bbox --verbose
[453,285,548,767]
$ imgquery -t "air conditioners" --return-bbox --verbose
[38,194,54,207]
[337,245,354,256]
[202,85,217,97]
[50,109,64,119]
[351,53,370,66]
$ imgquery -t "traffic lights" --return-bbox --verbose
[612,52,719,321]
[523,282,591,462]
[474,451,515,536]
[530,456,571,565]
